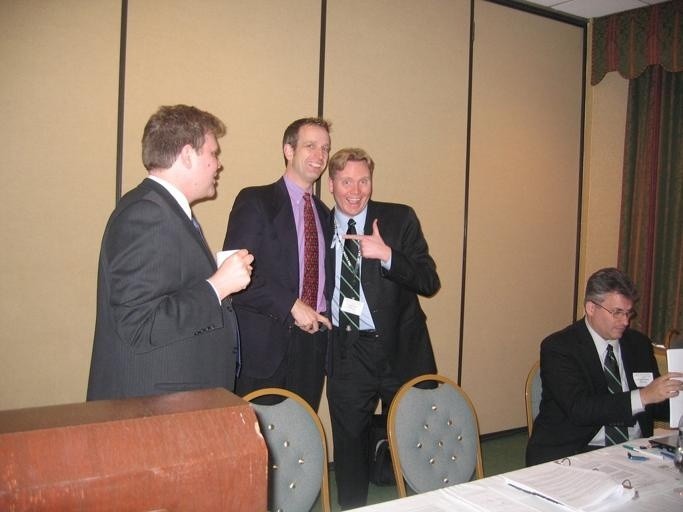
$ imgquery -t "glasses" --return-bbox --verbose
[587,298,636,321]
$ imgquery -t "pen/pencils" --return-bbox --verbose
[622,444,663,461]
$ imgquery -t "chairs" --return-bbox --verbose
[652,345,681,436]
[520,341,544,463]
[241,383,335,507]
[384,372,486,497]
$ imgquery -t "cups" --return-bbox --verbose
[216,249,242,270]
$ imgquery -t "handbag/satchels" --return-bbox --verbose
[368,415,396,488]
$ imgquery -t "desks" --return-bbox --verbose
[338,425,683,508]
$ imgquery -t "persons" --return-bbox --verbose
[221,115,333,415]
[326,145,441,512]
[85,103,255,395]
[525,267,683,470]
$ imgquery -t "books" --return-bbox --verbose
[501,456,639,512]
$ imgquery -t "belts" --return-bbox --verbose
[358,330,381,339]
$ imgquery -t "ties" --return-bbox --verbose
[340,219,359,332]
[302,193,319,314]
[603,344,629,449]
